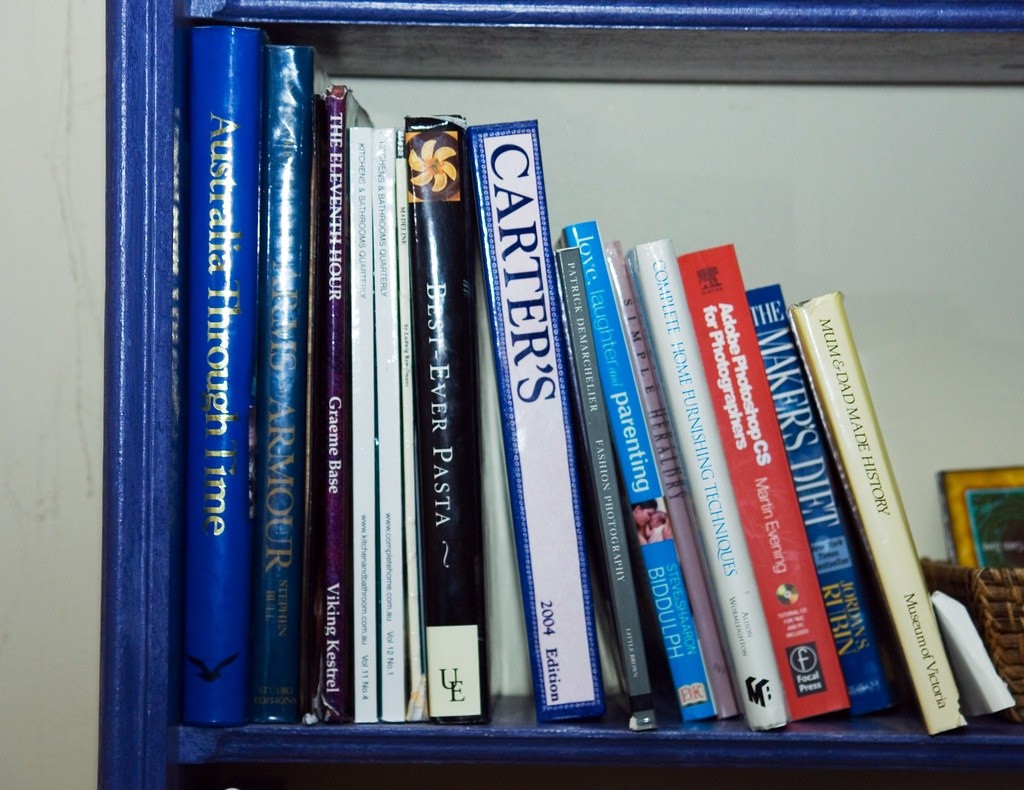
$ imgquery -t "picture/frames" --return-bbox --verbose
[935,463,1024,572]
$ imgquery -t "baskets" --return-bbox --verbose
[922,556,1024,721]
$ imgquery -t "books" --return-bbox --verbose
[173,17,971,740]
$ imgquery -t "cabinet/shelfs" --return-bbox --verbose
[103,0,1024,787]
